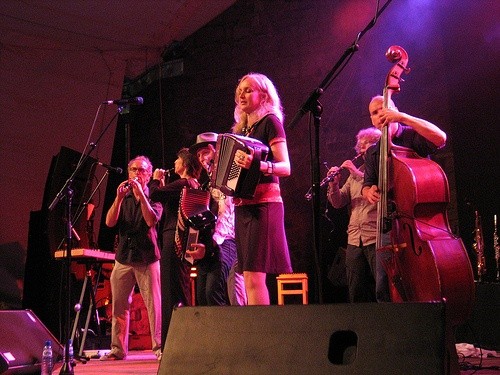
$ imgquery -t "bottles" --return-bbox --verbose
[41,341,53,375]
[63,339,73,363]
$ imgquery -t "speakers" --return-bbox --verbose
[456,282,500,351]
[157,300,461,375]
[0,308,64,375]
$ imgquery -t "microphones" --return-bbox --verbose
[98,162,122,174]
[102,97,143,105]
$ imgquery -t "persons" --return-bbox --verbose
[185,132,237,306]
[208,73,293,305]
[149,148,203,359]
[361,96,446,303]
[99,156,163,361]
[327,127,382,303]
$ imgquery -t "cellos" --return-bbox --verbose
[372,43,477,329]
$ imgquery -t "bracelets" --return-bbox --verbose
[264,161,272,176]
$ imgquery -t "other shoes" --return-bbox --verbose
[100,353,123,361]
[155,348,162,361]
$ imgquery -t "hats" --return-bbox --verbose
[189,132,218,156]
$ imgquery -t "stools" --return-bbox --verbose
[275,273,308,306]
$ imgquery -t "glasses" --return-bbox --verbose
[128,167,150,174]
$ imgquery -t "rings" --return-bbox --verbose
[240,155,244,159]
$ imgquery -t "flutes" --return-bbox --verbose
[304,151,365,201]
[122,176,139,193]
[150,167,175,178]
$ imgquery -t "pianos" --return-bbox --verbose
[53,244,117,260]
